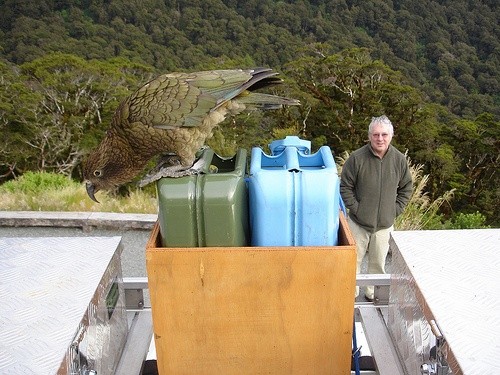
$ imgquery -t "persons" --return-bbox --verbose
[340,114,412,302]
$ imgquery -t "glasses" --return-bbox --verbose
[373,132,387,138]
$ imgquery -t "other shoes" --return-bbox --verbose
[365,289,375,302]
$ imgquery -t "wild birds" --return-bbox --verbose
[83,66,302,203]
